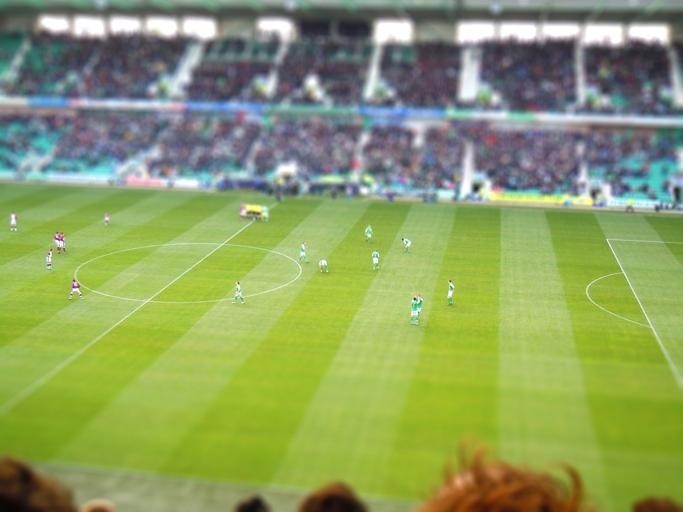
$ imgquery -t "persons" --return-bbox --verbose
[629,494,682,512]
[54,232,60,254]
[82,498,116,512]
[411,295,423,325]
[298,478,371,512]
[232,282,245,304]
[9,212,17,231]
[372,250,380,270]
[447,280,455,305]
[1,27,683,213]
[319,259,329,273]
[232,491,274,512]
[366,224,373,239]
[0,453,81,512]
[415,433,602,512]
[45,249,53,270]
[104,212,110,226]
[402,238,411,252]
[300,241,307,261]
[59,232,65,252]
[68,280,82,300]
